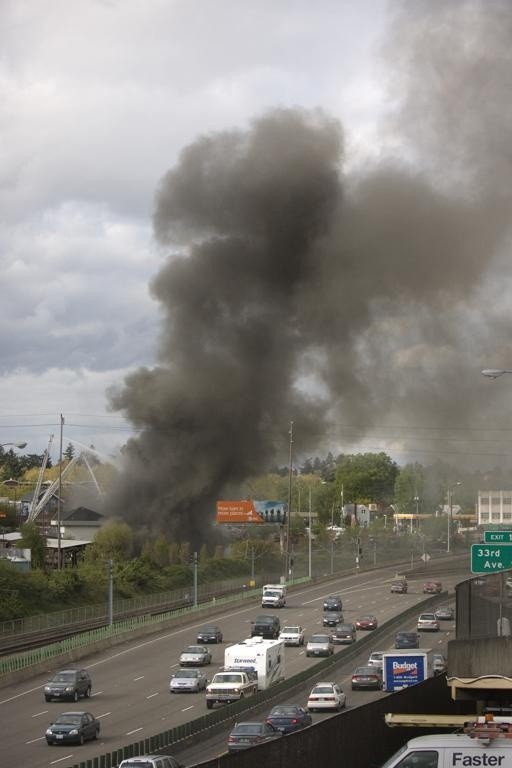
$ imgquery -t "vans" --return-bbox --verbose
[382,712,512,767]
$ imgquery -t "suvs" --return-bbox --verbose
[43,668,91,703]
[249,614,279,638]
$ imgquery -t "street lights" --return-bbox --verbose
[0,441,30,448]
[307,480,327,576]
[414,496,420,514]
[7,485,17,517]
[446,479,462,553]
[480,369,511,380]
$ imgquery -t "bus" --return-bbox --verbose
[393,513,479,533]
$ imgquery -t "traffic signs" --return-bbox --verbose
[484,530,512,543]
[470,543,512,575]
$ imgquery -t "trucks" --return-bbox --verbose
[261,584,287,609]
[206,638,285,709]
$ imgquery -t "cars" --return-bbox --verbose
[44,711,102,745]
[226,719,284,753]
[197,625,223,644]
[265,705,311,733]
[278,577,455,713]
[170,667,206,694]
[119,753,184,767]
[179,646,212,667]
